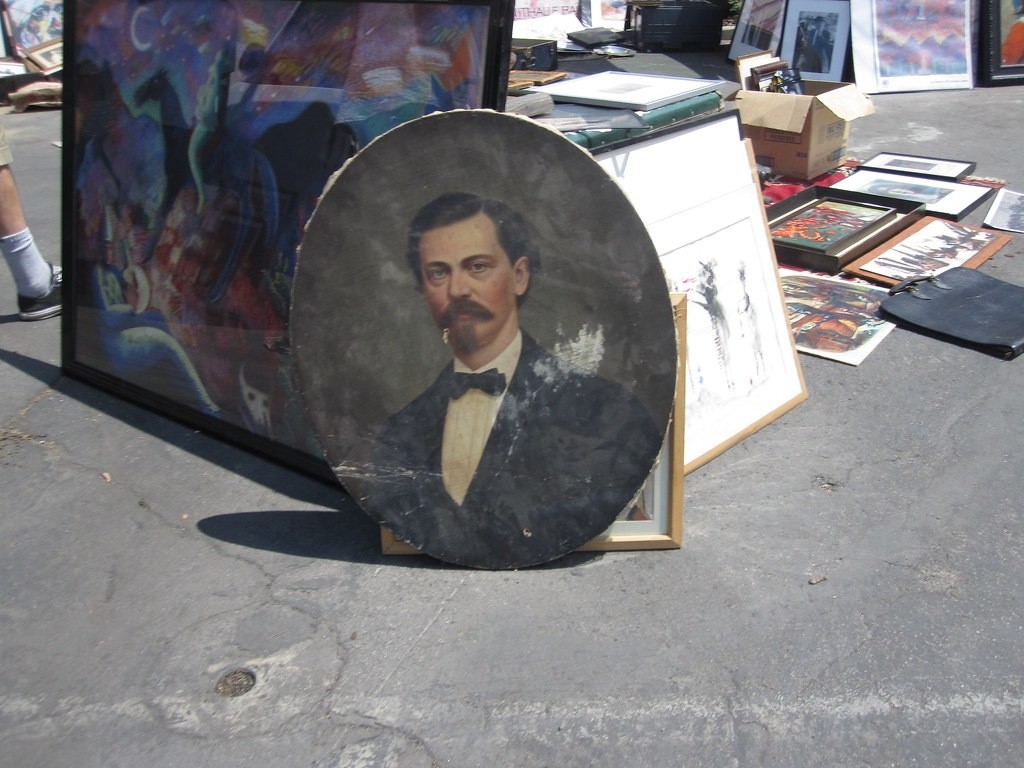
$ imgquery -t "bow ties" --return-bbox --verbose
[449,367,507,398]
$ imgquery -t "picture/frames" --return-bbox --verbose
[0,0,1018,574]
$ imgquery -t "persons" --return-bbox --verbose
[1,121,61,323]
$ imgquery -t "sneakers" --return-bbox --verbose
[17,261,63,322]
